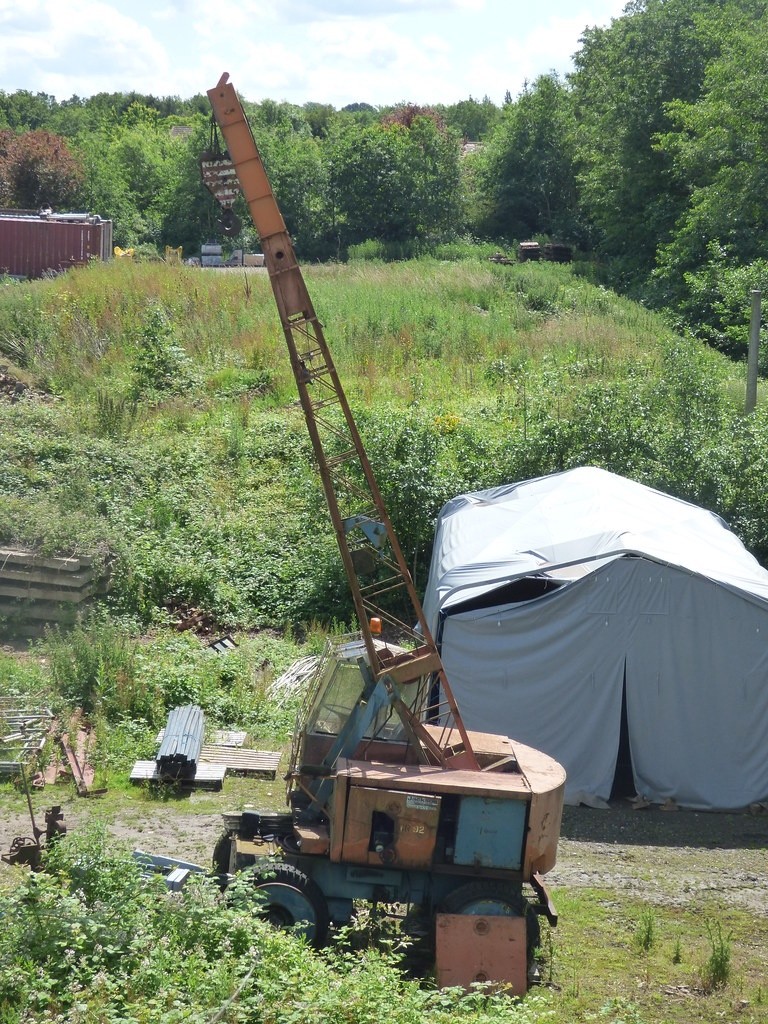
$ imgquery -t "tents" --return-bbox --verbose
[412,465,767,811]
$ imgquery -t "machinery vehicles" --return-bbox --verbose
[196,72,570,985]
